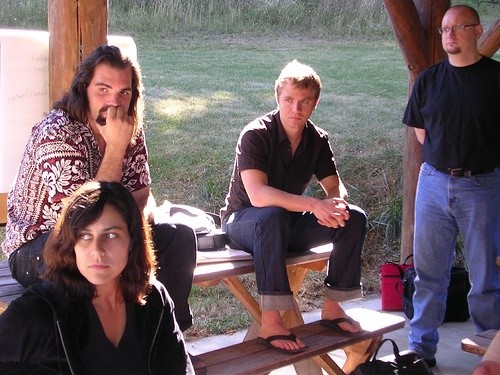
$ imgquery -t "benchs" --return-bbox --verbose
[180,309,406,375]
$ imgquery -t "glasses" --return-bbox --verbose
[438,22,481,34]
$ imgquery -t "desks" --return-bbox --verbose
[0,242,335,375]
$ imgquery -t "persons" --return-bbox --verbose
[0,179,197,375]
[393,5,500,366]
[0,45,208,375]
[220,59,367,353]
[473,329,500,375]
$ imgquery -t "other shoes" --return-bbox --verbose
[424,357,437,368]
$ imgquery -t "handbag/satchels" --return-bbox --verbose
[396,267,471,323]
[349,338,435,375]
[380,254,413,311]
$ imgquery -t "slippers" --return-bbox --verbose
[257,334,306,354]
[320,316,363,336]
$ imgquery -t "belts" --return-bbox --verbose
[435,165,499,177]
